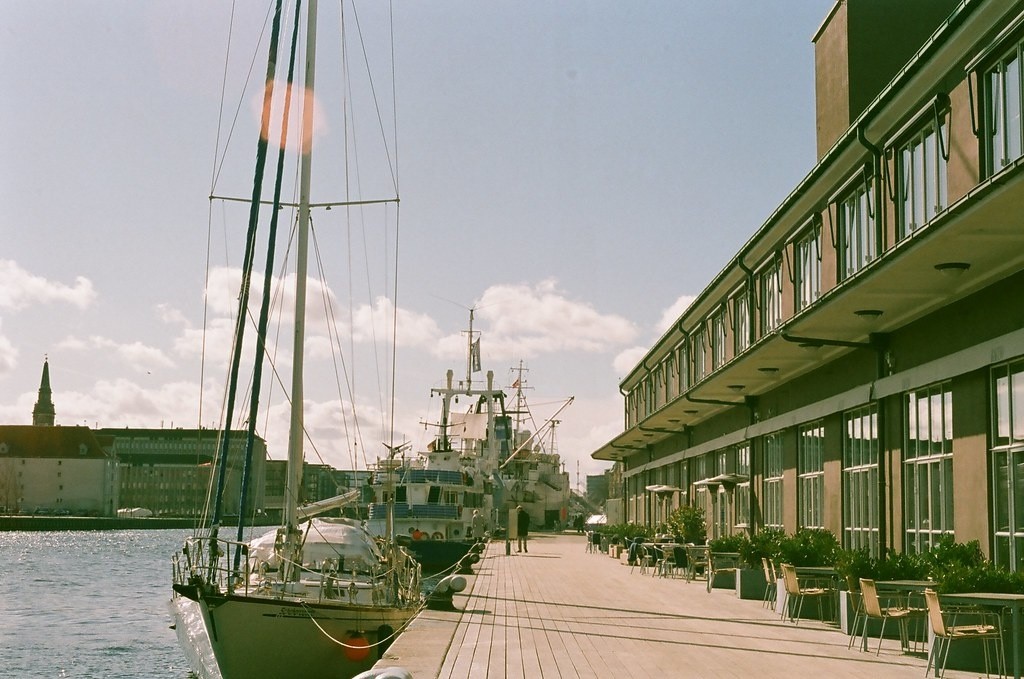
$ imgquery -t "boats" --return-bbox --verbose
[357,299,501,571]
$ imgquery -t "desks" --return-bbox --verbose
[863,579,942,652]
[712,551,741,586]
[790,566,840,623]
[641,542,676,546]
[682,544,710,549]
[935,591,1024,679]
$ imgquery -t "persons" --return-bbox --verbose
[409,527,421,539]
[577,513,584,533]
[472,510,485,543]
[517,505,530,553]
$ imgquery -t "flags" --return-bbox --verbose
[512,380,518,388]
[472,338,481,372]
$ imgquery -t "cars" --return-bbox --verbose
[18,509,28,515]
[255,508,269,518]
[35,507,99,517]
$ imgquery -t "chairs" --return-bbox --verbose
[783,563,826,625]
[768,557,778,612]
[585,531,744,592]
[758,556,773,609]
[779,562,819,623]
[846,576,1007,679]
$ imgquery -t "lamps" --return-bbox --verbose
[797,343,826,353]
[759,367,780,374]
[852,308,885,322]
[606,419,680,460]
[934,261,971,276]
[682,410,699,417]
[727,384,746,392]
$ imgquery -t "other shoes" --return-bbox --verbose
[516,551,522,552]
[525,551,528,553]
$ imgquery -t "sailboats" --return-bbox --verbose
[170,1,429,679]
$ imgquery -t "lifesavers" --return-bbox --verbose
[431,531,444,540]
[419,532,430,541]
